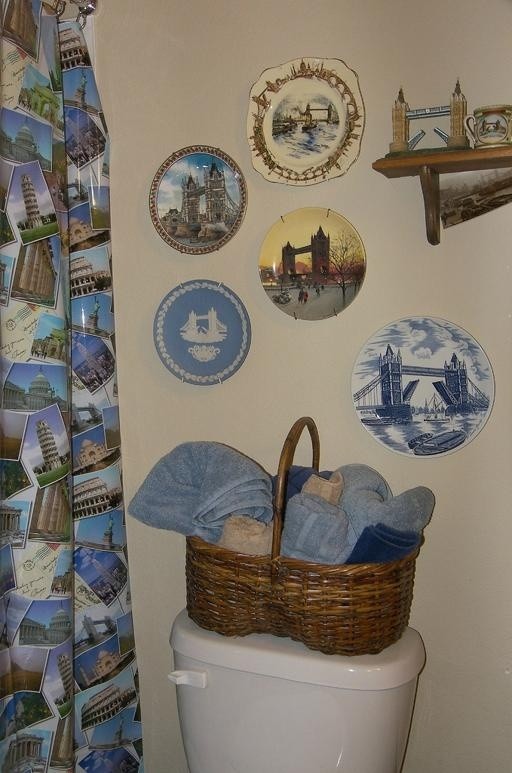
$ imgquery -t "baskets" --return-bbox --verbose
[185,418,425,659]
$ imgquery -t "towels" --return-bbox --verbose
[127,441,435,565]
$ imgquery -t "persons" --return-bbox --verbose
[297,280,324,304]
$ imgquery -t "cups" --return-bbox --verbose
[464,104,511,149]
